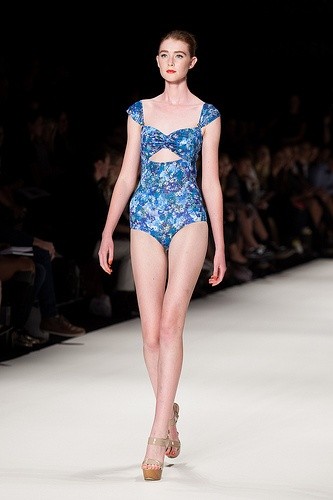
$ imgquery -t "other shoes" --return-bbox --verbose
[229,255,247,265]
[39,316,86,336]
[8,328,45,346]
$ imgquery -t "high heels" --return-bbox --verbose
[165,403,181,458]
[141,437,169,480]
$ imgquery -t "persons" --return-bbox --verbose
[0,59,333,356]
[97,30,226,481]
